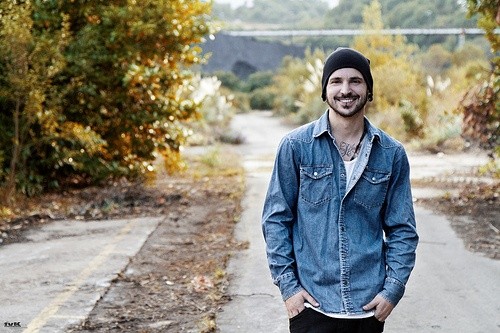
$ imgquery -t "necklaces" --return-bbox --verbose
[350,121,368,162]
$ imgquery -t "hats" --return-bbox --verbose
[321,46,374,103]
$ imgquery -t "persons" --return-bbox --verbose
[259,47,420,333]
